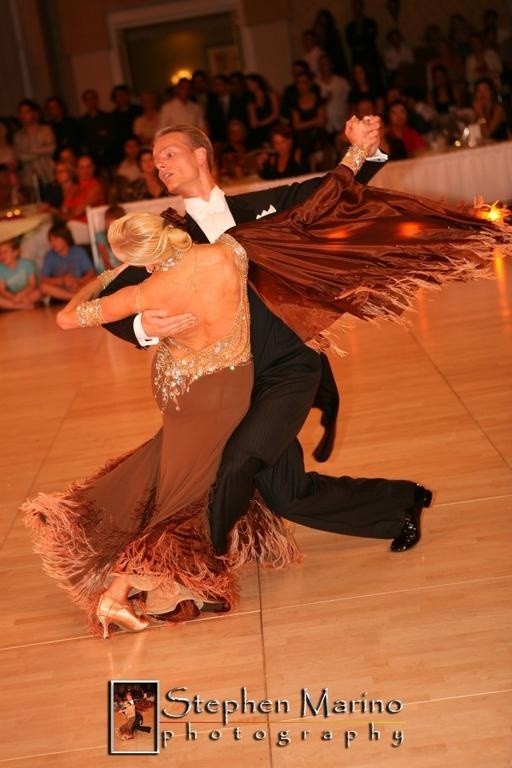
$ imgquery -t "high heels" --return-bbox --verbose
[95,592,152,640]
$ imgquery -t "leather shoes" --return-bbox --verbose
[389,482,433,553]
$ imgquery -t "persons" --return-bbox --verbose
[116,692,152,742]
[18,113,512,640]
[96,118,434,555]
[1,0,512,315]
